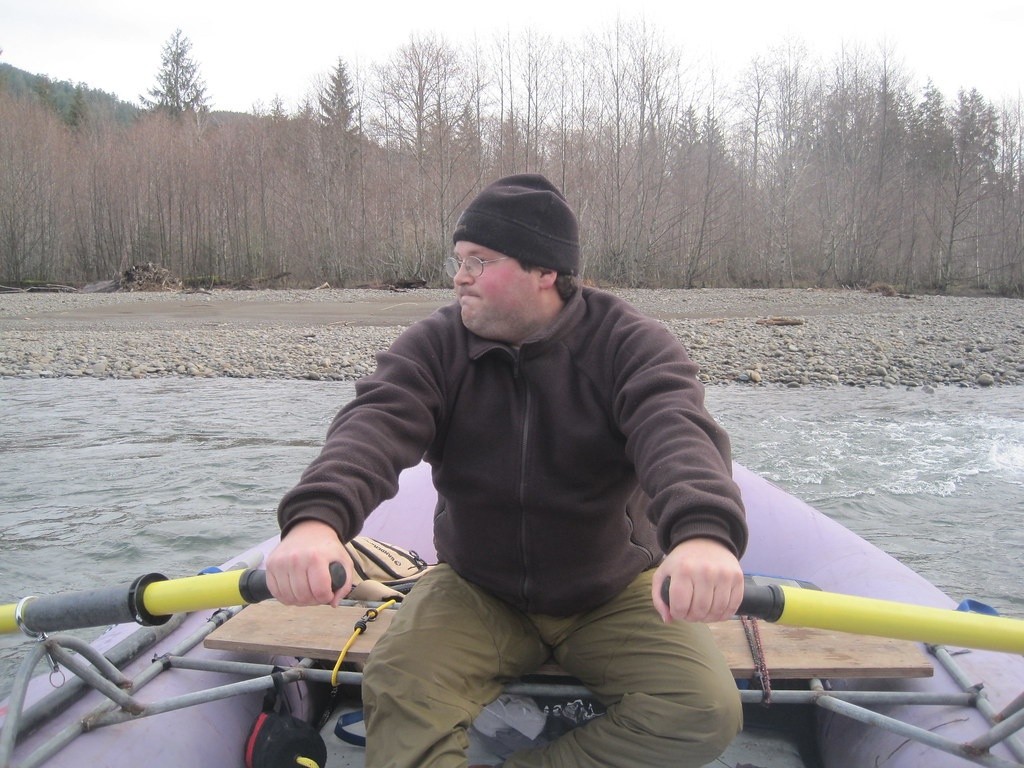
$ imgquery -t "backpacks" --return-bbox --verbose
[344,535,435,602]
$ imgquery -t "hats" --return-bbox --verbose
[452,172,581,274]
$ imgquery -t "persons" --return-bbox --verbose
[261,174,747,767]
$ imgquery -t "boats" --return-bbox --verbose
[0,448,1024,768]
